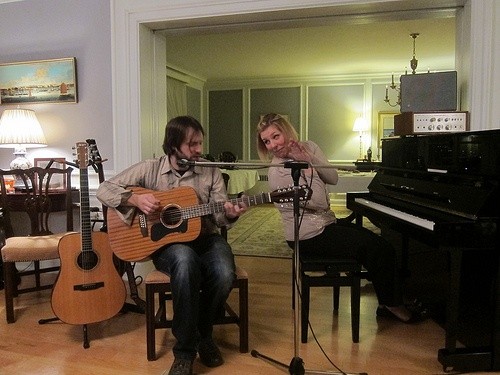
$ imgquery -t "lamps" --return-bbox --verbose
[353,117,369,162]
[385,32,429,108]
[0,104,48,187]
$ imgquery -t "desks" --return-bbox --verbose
[219,168,260,198]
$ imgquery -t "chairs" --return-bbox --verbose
[206,150,237,170]
[144,173,248,360]
[0,167,73,323]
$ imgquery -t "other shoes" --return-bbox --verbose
[198,347,224,367]
[376,306,426,323]
[167,357,194,375]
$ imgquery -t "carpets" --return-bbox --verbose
[226,206,381,259]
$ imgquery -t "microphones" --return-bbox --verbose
[178,159,188,168]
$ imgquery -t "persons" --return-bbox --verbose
[97,115,249,375]
[256,112,428,324]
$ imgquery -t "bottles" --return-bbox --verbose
[367,148,372,160]
[2,168,15,194]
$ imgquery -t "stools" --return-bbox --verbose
[292,252,362,343]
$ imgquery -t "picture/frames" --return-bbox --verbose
[34,158,67,193]
[377,111,400,161]
[0,56,78,106]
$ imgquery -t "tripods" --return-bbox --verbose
[177,160,372,375]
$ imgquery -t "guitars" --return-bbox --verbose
[106,184,313,263]
[49,141,128,326]
[85,138,128,279]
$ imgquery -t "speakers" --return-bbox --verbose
[400,71,457,112]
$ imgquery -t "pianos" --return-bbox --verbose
[346,129,500,374]
